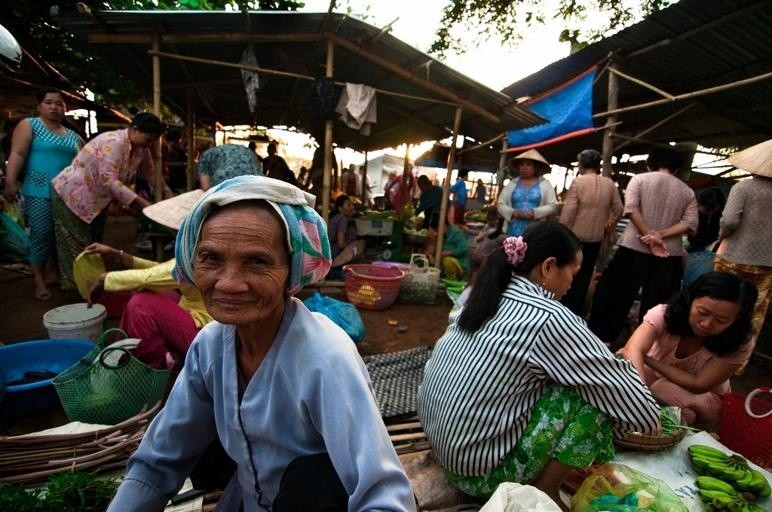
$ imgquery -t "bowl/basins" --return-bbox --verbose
[1,342,97,393]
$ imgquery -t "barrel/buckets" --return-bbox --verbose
[42,302,106,345]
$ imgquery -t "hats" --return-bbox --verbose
[141,189,206,230]
[729,138,771,177]
[507,148,552,176]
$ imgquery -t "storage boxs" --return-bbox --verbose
[355,218,393,236]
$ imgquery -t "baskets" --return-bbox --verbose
[342,264,406,311]
[49,328,172,425]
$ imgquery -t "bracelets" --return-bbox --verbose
[119,248,124,265]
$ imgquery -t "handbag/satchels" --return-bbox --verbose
[399,252,440,305]
[721,386,772,469]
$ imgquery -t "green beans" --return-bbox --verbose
[659,408,700,434]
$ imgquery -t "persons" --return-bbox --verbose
[387,163,417,211]
[615,269,757,429]
[589,148,699,352]
[6,88,86,303]
[50,112,163,304]
[327,194,357,260]
[431,217,470,281]
[415,175,449,228]
[496,149,557,238]
[262,143,289,181]
[449,170,469,225]
[105,176,417,512]
[85,189,214,368]
[197,143,261,191]
[712,138,772,379]
[558,148,623,320]
[416,220,663,512]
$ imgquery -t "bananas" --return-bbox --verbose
[694,475,772,512]
[687,445,772,501]
